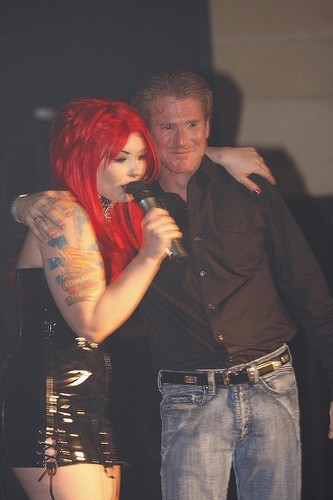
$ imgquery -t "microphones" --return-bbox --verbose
[126,181,189,265]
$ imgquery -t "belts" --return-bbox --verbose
[161,349,290,385]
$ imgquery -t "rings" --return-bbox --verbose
[33,215,42,220]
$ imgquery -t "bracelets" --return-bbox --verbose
[9,192,34,227]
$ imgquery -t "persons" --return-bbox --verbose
[1,97,276,499]
[4,68,333,500]
